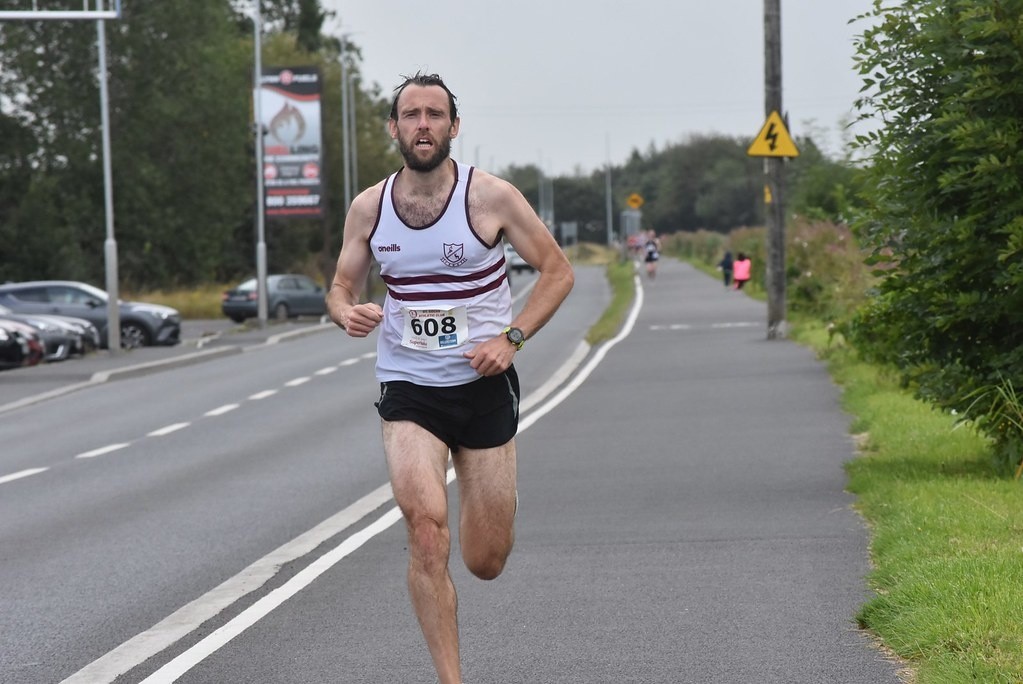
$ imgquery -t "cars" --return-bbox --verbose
[504,242,537,274]
[0,304,101,368]
[0,280,182,350]
[221,274,331,323]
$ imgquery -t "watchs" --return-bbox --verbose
[503,324,527,350]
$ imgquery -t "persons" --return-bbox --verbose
[643,229,662,277]
[716,251,733,286]
[732,252,751,290]
[325,69,575,684]
